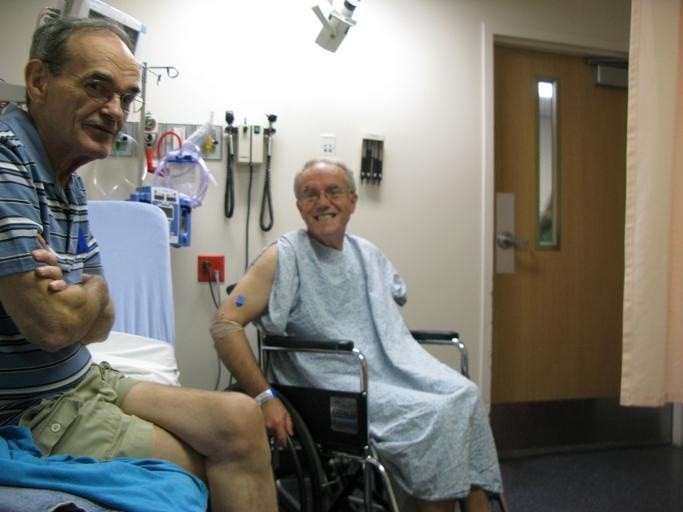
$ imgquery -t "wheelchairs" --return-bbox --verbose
[219,282,513,512]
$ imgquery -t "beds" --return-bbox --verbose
[1,202,177,510]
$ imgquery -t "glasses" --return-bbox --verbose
[65,70,145,115]
[294,184,350,199]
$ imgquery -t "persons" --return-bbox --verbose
[0,16,281,512]
[208,155,504,511]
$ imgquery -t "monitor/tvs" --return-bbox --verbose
[62,0,144,55]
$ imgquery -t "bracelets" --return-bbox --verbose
[252,387,277,406]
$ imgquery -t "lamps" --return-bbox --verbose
[310,0,364,55]
